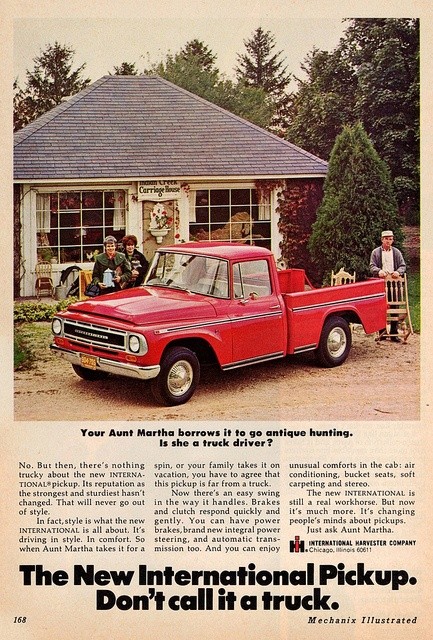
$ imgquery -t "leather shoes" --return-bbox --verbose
[381,337,385,340]
[391,337,400,342]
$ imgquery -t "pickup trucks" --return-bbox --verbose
[49,240,386,407]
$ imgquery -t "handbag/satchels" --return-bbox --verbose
[84,277,100,297]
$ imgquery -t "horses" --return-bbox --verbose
[196,211,264,245]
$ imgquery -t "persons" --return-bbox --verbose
[91,236,131,294]
[120,234,149,287]
[369,230,407,343]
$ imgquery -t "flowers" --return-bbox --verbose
[176,234,186,243]
[153,211,173,228]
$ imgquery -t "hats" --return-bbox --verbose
[381,230,394,238]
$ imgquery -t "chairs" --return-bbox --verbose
[374,274,414,345]
[34,261,55,299]
[331,268,356,339]
